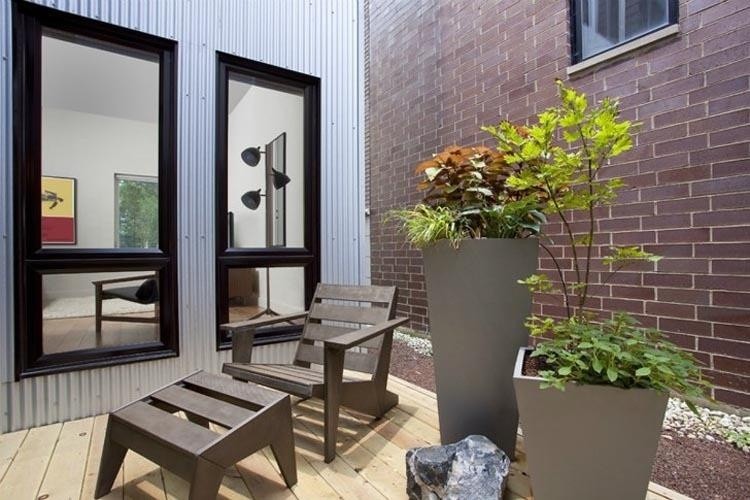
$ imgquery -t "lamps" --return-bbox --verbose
[241,145,296,326]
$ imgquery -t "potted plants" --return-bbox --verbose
[477,79,722,500]
[389,128,539,462]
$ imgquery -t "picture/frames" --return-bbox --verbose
[41,174,76,245]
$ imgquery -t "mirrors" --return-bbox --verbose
[265,131,286,248]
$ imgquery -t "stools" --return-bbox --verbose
[95,370,297,500]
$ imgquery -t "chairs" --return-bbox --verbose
[93,267,160,333]
[218,283,409,463]
